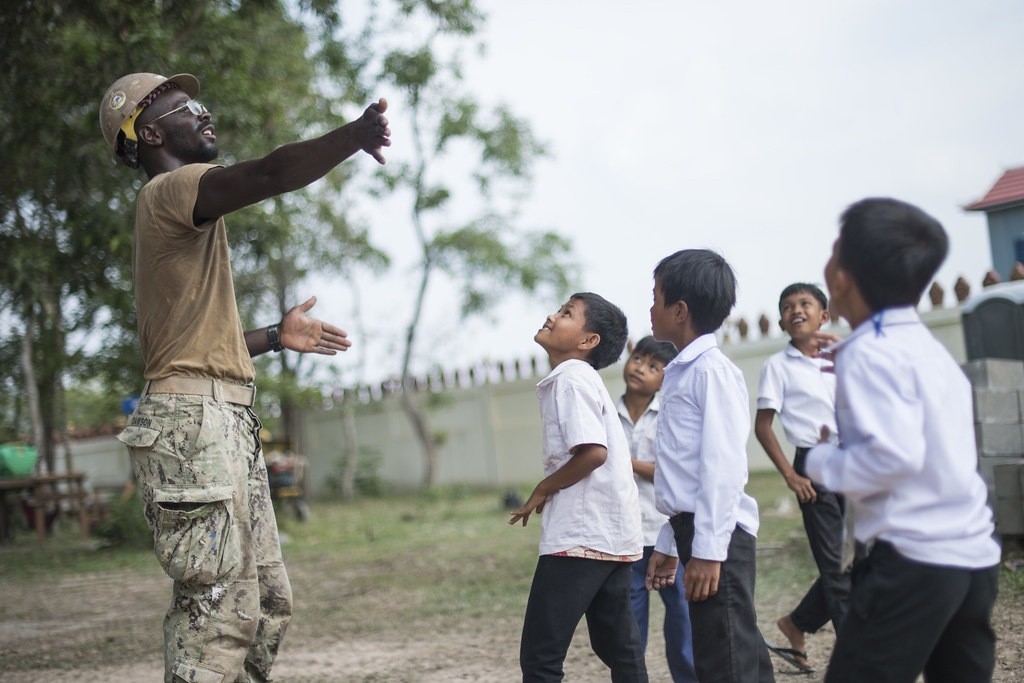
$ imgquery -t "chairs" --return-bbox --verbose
[20,451,90,543]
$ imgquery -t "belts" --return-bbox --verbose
[148,376,257,407]
[795,448,809,456]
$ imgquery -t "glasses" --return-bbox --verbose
[138,99,212,142]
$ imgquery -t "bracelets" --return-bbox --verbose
[267,324,282,352]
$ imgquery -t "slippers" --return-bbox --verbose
[766,642,817,672]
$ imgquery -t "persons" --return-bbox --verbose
[99,71,392,683]
[510,291,649,683]
[616,335,696,683]
[645,248,775,683]
[803,195,1002,683]
[754,285,851,670]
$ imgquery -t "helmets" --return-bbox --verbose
[99,73,201,164]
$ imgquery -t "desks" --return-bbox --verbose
[0,470,89,539]
